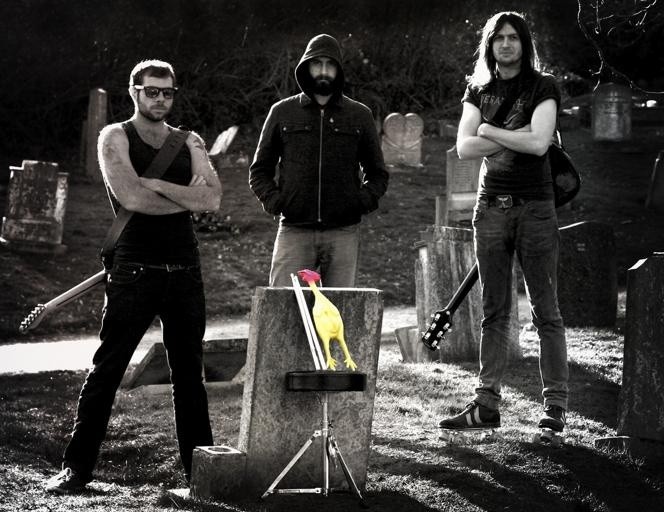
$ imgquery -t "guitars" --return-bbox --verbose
[19,269,107,331]
[419,145,581,350]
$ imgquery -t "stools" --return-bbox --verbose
[256,369,366,503]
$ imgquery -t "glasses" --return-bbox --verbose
[134,84,179,99]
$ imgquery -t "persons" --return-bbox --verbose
[248,34,389,287]
[439,11,569,430]
[46,61,222,494]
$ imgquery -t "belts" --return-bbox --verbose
[147,262,184,272]
[486,193,529,207]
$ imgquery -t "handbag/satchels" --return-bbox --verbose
[549,142,580,209]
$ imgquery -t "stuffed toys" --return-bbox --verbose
[297,269,357,371]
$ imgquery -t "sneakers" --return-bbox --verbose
[45,468,91,492]
[538,405,566,432]
[439,403,501,429]
